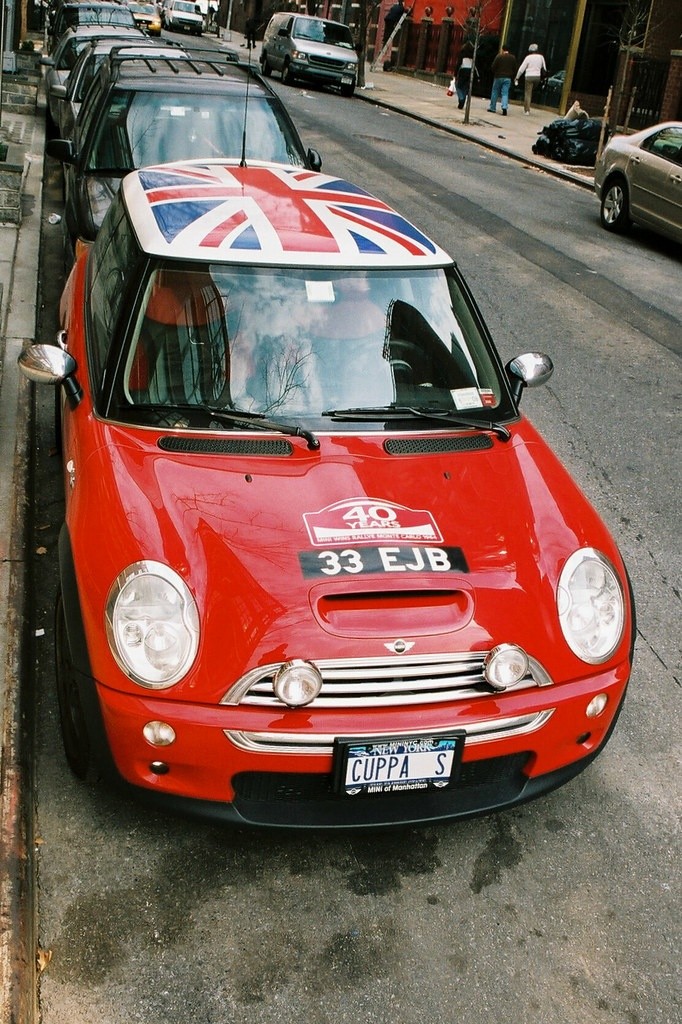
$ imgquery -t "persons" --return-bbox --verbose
[514,44,547,115]
[487,44,517,116]
[454,47,480,110]
[209,4,216,26]
[245,17,256,49]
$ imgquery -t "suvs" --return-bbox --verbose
[44,44,322,272]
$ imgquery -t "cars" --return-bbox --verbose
[18,162,639,832]
[40,0,205,142]
[594,119,682,242]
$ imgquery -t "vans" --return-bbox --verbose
[259,12,359,98]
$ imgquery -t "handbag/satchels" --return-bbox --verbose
[447,77,456,96]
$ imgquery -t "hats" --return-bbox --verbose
[528,44,538,51]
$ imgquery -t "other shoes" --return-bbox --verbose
[523,110,529,114]
[458,100,463,108]
[487,109,495,112]
[503,109,506,115]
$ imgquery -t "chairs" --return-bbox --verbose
[130,278,391,405]
